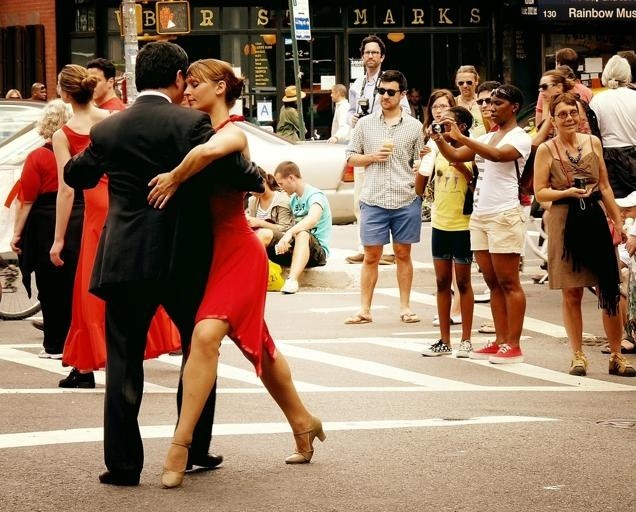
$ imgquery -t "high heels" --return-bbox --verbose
[287,417,325,465]
[160,439,188,489]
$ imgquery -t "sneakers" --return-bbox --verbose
[346,252,397,265]
[608,353,636,377]
[38,349,63,359]
[281,278,300,295]
[56,367,97,388]
[569,352,589,375]
[424,308,525,366]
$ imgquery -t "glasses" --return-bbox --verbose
[457,80,474,86]
[377,87,402,96]
[539,83,557,90]
[555,109,579,118]
[490,88,511,98]
[475,97,491,105]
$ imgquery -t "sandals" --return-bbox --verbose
[602,333,636,355]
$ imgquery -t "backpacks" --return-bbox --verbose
[519,145,538,206]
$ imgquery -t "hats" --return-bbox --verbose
[282,84,307,103]
[615,189,636,209]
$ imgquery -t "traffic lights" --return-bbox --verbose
[156,2,186,33]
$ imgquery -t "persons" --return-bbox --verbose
[85,58,128,110]
[245,166,293,232]
[10,99,84,360]
[63,40,264,487]
[277,85,308,141]
[253,160,332,293]
[146,58,326,488]
[6,89,22,99]
[49,63,182,389]
[28,82,46,100]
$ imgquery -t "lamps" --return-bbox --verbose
[387,32,405,43]
[261,34,277,46]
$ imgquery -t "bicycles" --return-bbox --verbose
[0,247,43,321]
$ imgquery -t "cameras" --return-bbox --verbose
[575,178,585,189]
[358,99,369,118]
[432,123,444,133]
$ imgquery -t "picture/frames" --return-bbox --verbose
[155,1,191,36]
[255,98,274,123]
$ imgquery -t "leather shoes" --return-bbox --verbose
[186,452,224,471]
[99,469,140,486]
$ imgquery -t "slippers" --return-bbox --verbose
[345,313,372,325]
[399,311,421,322]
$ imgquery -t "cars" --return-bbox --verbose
[0,98,50,148]
